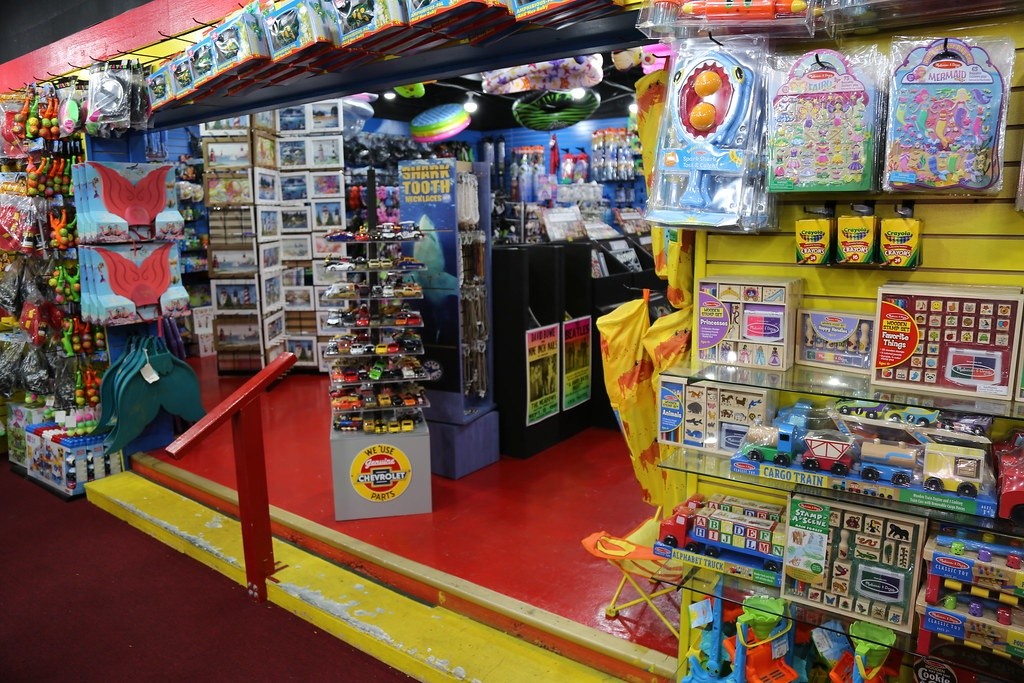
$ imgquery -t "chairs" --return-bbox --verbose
[581,505,679,640]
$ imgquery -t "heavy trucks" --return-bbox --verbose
[658,493,787,577]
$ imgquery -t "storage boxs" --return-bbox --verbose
[329,408,433,522]
[188,305,212,335]
[144,60,178,113]
[698,273,805,372]
[6,399,122,495]
[325,0,409,48]
[657,374,770,457]
[208,0,271,77]
[780,493,927,634]
[511,0,587,23]
[191,334,218,358]
[187,27,228,90]
[166,49,209,103]
[405,0,489,30]
[793,306,875,376]
[261,0,333,65]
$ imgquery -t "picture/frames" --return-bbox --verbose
[260,270,286,314]
[258,240,282,275]
[311,232,347,259]
[313,286,349,311]
[316,312,350,336]
[267,344,286,365]
[287,335,318,367]
[281,206,312,233]
[212,317,260,352]
[210,279,260,315]
[263,309,286,349]
[281,234,313,261]
[310,197,347,231]
[283,286,315,311]
[198,99,345,207]
[256,205,283,243]
[316,342,332,372]
[311,259,346,286]
[207,244,256,279]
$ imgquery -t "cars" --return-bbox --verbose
[323,219,434,431]
[744,401,993,500]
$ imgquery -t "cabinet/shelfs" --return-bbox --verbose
[650,355,1024,683]
[322,237,431,434]
